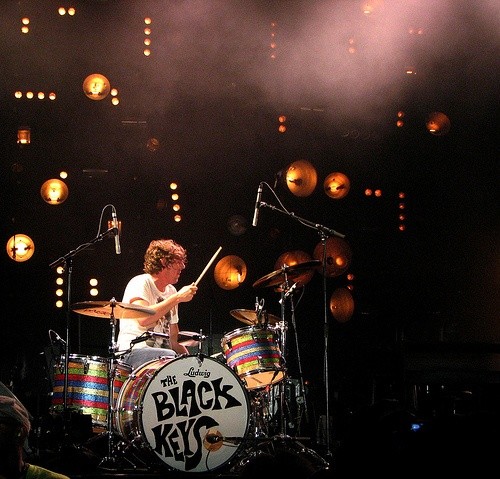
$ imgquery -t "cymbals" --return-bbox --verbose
[229,309,281,325]
[71,300,156,319]
[253,259,322,288]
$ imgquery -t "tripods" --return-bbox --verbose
[232,278,331,472]
[96,306,139,470]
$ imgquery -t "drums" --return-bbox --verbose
[221,325,288,389]
[51,352,134,433]
[115,352,251,473]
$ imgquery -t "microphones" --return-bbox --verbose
[56,333,65,345]
[279,282,297,304]
[112,207,122,255]
[147,331,169,338]
[252,185,263,227]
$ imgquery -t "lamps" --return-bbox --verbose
[276,159,318,198]
[324,172,352,199]
[39,178,70,212]
[214,237,355,323]
[5,233,36,263]
[83,70,110,100]
[426,111,450,136]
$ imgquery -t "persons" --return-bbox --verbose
[241,452,313,479]
[117,239,198,372]
[0,395,70,479]
